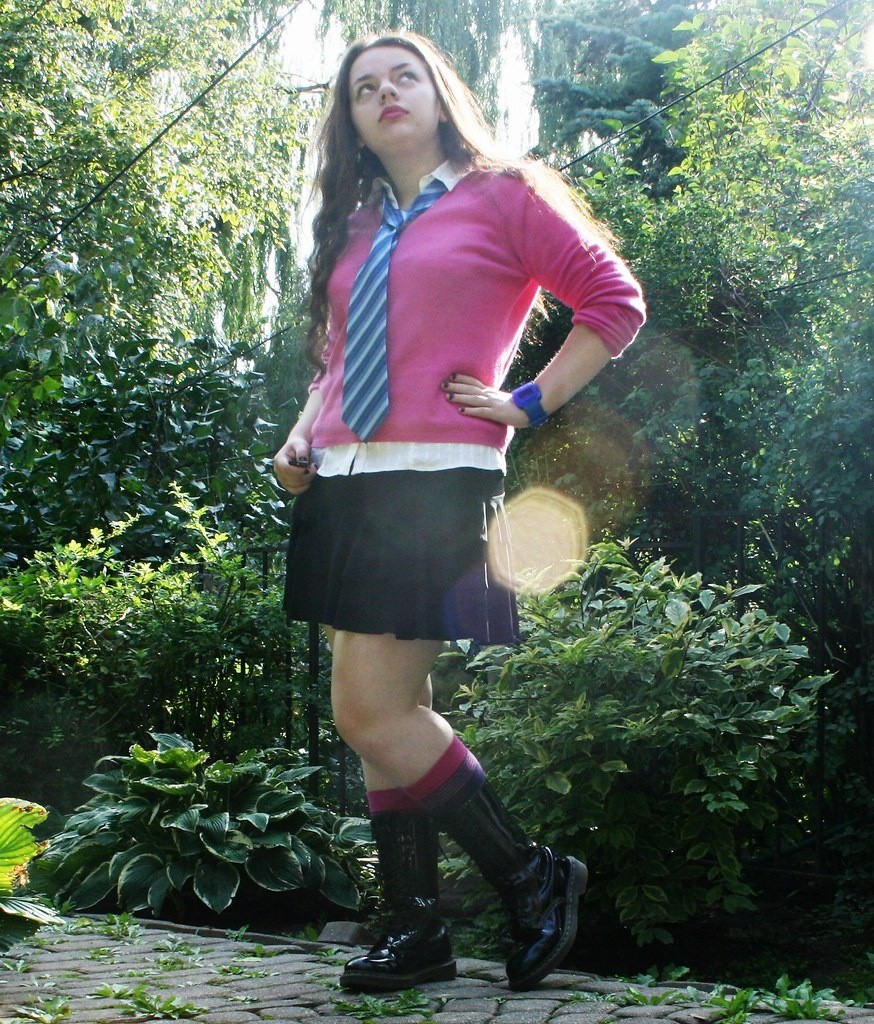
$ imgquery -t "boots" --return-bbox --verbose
[440,776,591,990]
[341,811,456,992]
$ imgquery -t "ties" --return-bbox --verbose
[340,178,448,443]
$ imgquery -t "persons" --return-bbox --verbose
[277,32,649,993]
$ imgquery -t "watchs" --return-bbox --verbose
[510,381,549,427]
